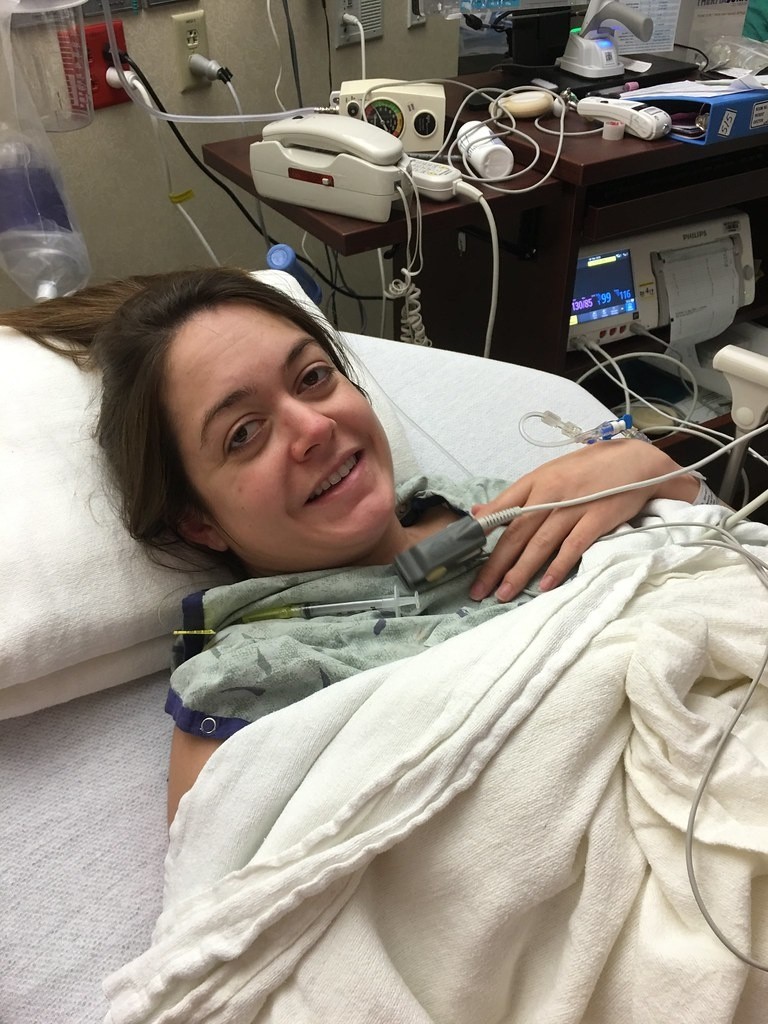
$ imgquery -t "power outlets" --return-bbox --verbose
[59,20,136,113]
[171,8,213,95]
[333,0,383,51]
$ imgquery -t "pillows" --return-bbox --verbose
[2,269,425,725]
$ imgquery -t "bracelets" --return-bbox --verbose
[689,470,720,507]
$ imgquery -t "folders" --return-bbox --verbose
[620,90,768,147]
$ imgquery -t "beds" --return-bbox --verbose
[0,267,624,1024]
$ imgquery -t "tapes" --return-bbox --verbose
[602,121,625,140]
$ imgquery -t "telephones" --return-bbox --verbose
[247,111,417,225]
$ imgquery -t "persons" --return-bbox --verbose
[2,263,751,843]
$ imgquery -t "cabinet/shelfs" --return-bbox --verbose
[202,70,768,451]
